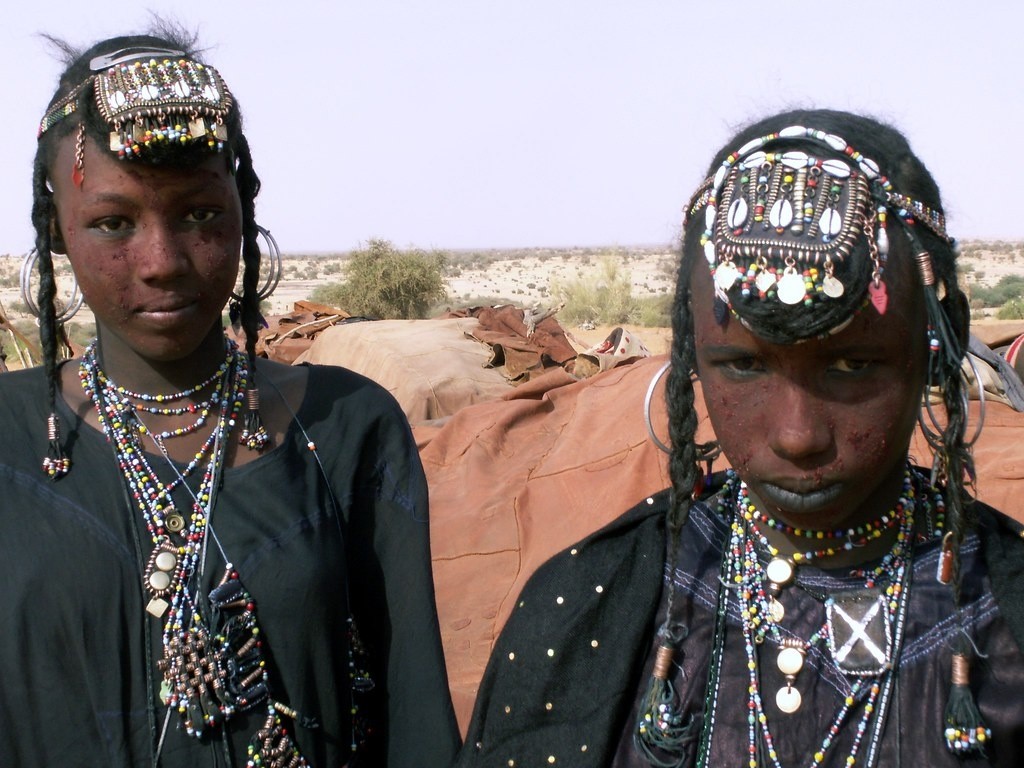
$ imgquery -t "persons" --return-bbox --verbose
[451,109,1024,768]
[0,31,465,768]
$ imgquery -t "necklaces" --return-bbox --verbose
[697,465,946,768]
[76,334,310,767]
